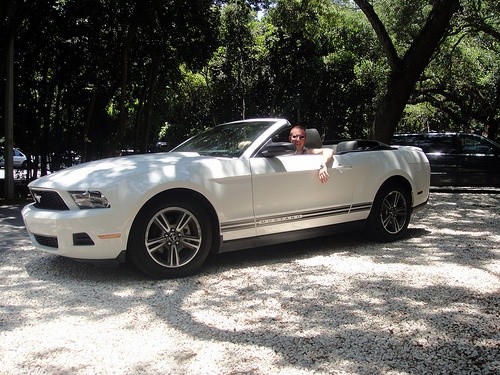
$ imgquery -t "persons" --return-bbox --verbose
[289,126,334,183]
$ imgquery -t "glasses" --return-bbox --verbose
[292,135,304,140]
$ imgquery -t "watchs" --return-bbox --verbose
[319,164,324,169]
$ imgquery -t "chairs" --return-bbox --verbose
[336,141,358,151]
[305,129,322,148]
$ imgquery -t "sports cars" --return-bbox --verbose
[21,117,431,280]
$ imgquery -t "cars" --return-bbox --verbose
[0,148,29,170]
[392,133,500,189]
